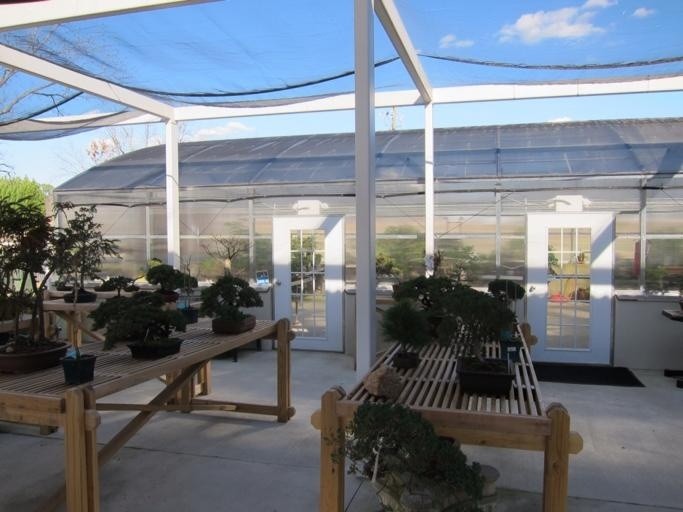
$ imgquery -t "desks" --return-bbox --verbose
[0,314,300,510]
[310,320,586,512]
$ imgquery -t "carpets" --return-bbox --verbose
[517,361,647,390]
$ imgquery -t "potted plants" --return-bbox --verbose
[2,169,142,390]
[87,261,208,360]
[199,262,263,334]
[375,251,542,410]
